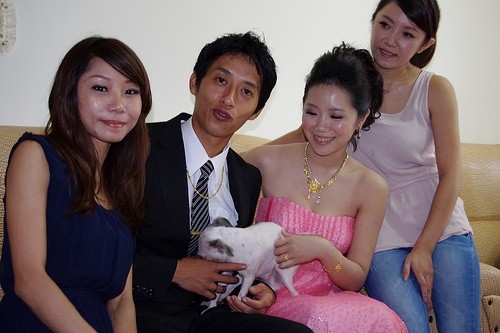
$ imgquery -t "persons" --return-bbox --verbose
[132,31,312,333]
[239,0,482,333]
[238,42,410,333]
[0,36,151,333]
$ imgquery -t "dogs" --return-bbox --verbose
[198,216,299,315]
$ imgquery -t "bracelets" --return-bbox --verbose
[322,251,344,273]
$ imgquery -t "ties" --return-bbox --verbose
[188,159,214,257]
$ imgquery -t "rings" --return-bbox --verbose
[284,253,288,260]
[214,283,218,291]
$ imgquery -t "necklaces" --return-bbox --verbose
[303,141,350,205]
[186,164,225,200]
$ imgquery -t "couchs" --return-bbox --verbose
[0,125,273,262]
[427,145,500,333]
